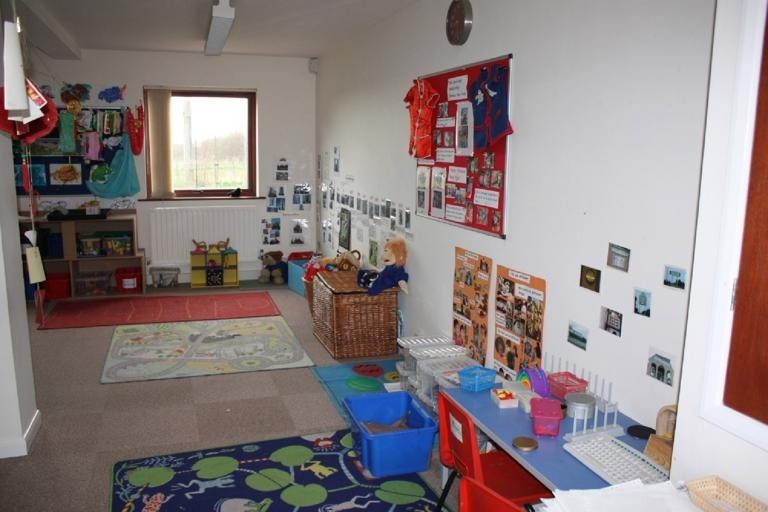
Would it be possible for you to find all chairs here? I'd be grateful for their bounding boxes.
[459,476,525,512]
[433,392,554,511]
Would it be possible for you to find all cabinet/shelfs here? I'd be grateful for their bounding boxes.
[18,208,147,300]
[13,107,123,156]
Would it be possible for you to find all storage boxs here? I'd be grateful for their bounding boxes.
[397,334,451,357]
[115,267,141,292]
[529,398,562,437]
[415,356,480,395]
[343,390,438,476]
[149,266,181,288]
[288,259,309,296]
[431,401,438,424]
[77,235,100,257]
[97,230,133,257]
[549,371,588,401]
[311,270,401,360]
[408,346,472,380]
[435,373,508,394]
[415,392,432,414]
[395,361,416,388]
[39,273,69,298]
[73,271,113,295]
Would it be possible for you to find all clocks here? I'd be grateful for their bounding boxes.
[446,0,472,45]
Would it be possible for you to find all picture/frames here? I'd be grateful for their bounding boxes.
[15,153,91,195]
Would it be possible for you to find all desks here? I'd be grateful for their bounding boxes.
[440,382,670,494]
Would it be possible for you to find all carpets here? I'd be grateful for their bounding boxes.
[109,425,452,512]
[100,314,316,383]
[37,290,281,330]
